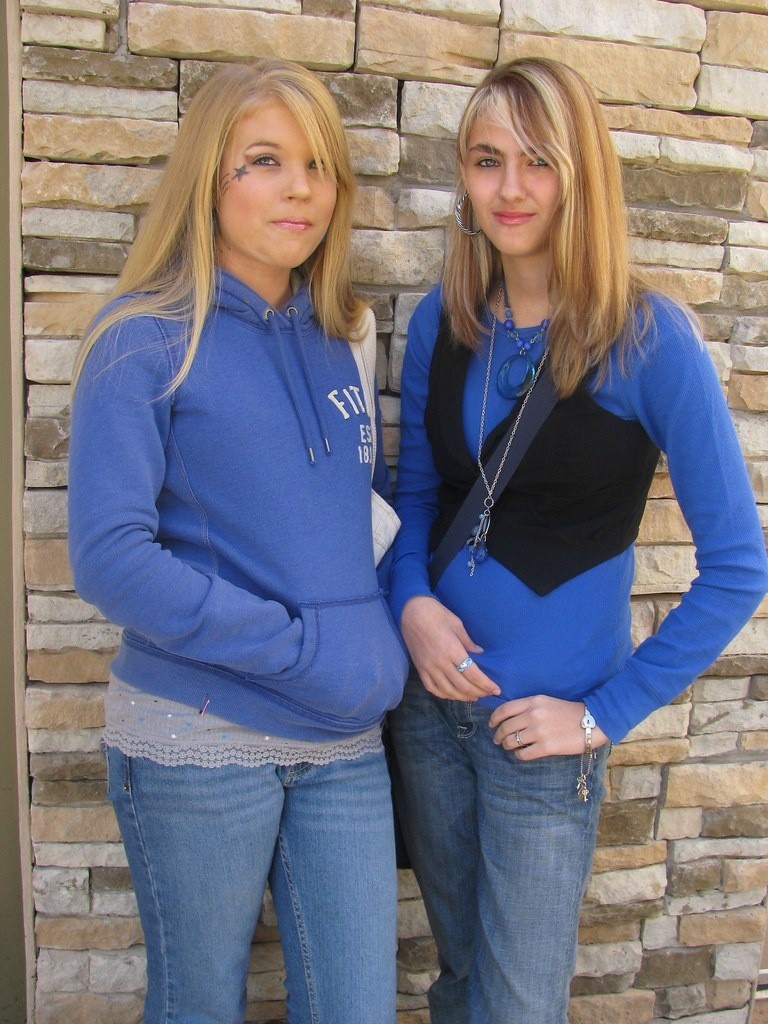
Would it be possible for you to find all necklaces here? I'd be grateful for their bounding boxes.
[467,282,549,577]
[497,264,549,400]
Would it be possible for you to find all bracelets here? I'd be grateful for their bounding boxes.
[577,705,595,802]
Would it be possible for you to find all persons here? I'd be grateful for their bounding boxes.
[387,58,768,1023]
[69,57,412,1024]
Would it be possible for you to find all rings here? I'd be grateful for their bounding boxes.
[457,658,472,672]
[516,732,526,746]
